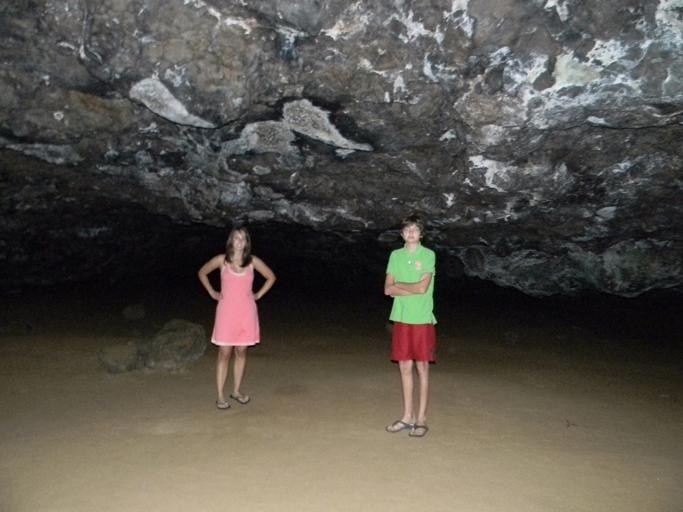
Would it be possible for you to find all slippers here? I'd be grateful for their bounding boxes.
[215,400,231,410]
[230,392,250,405]
[386,419,413,433]
[408,422,429,437]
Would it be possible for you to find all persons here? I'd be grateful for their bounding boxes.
[384,215,438,437]
[197,226,276,409]
